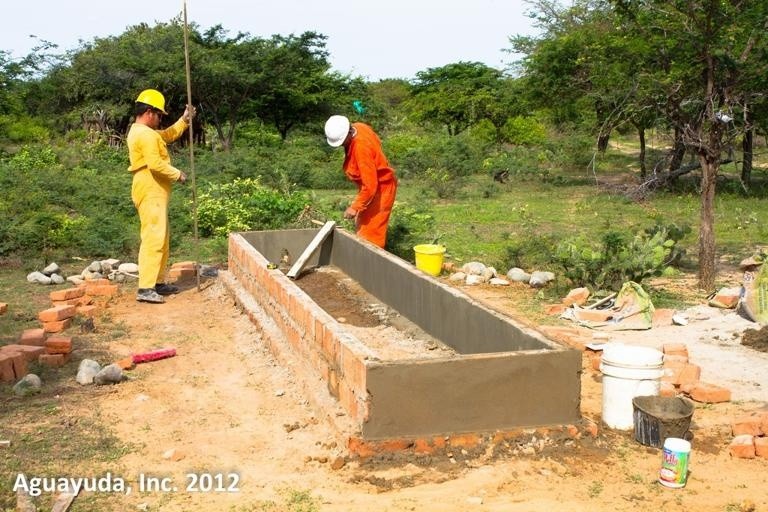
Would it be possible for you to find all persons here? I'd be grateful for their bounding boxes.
[325,115,398,248]
[126,88,197,303]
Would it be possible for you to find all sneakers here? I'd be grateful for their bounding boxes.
[136,283,180,303]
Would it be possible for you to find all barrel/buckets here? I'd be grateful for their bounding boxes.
[412,243,447,275]
[631,396,695,447]
[599,344,665,428]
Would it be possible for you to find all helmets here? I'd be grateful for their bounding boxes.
[325,114,350,148]
[136,89,169,115]
